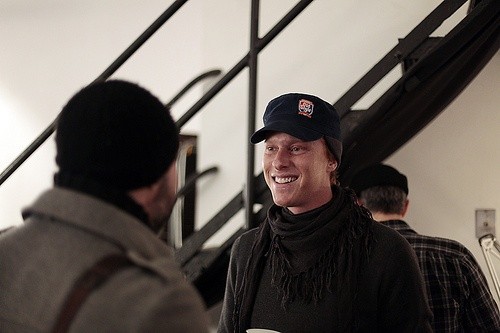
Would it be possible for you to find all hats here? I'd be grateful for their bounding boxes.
[55,79,178,190]
[351,163,408,195]
[250,93,342,141]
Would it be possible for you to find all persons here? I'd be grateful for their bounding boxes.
[0,80,210,333]
[354,164,500,333]
[217,93,435,333]
